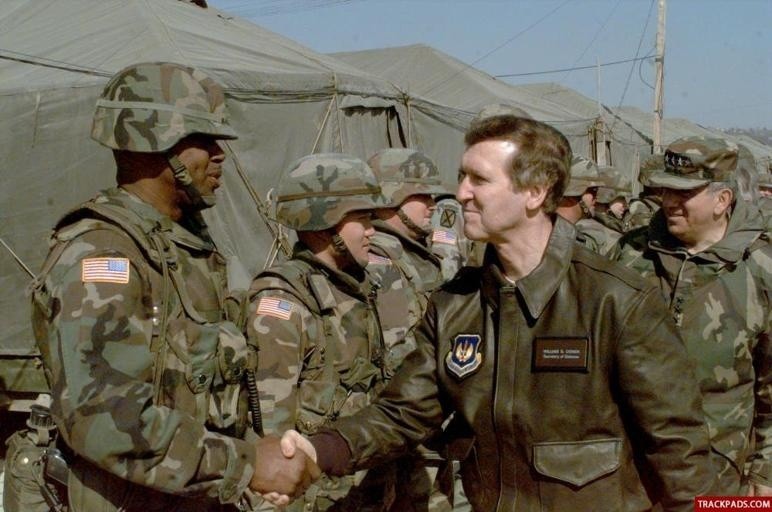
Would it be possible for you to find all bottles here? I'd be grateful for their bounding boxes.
[3,405,63,512]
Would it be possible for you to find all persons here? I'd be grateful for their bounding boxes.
[29,60,322,512]
[252,111,717,511]
[248,151,402,512]
[603,132,771,511]
[366,146,772,511]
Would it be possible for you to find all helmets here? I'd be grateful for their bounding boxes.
[368,149,446,208]
[91,61,238,154]
[563,145,771,203]
[274,153,386,231]
